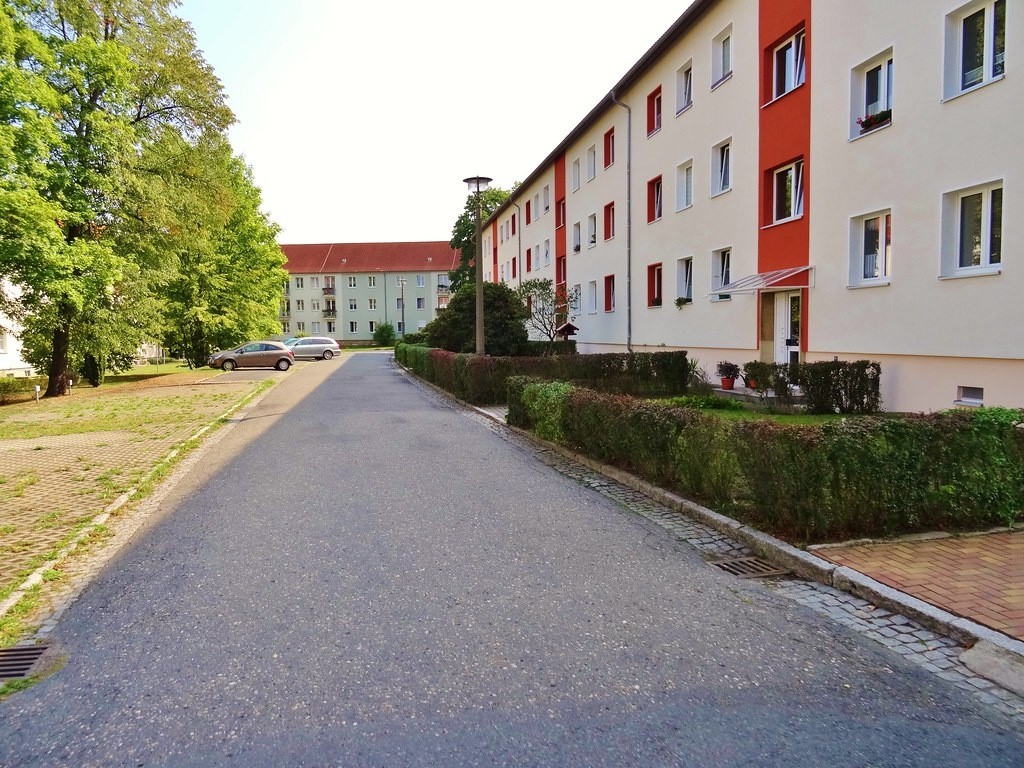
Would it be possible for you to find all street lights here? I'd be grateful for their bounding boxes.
[463,175,494,357]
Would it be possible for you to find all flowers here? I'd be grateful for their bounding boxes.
[854,108,892,130]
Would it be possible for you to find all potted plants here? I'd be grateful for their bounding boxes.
[714,360,770,391]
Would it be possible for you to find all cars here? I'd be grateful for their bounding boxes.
[287,336,341,360]
[207,340,295,371]
[268,338,304,350]
